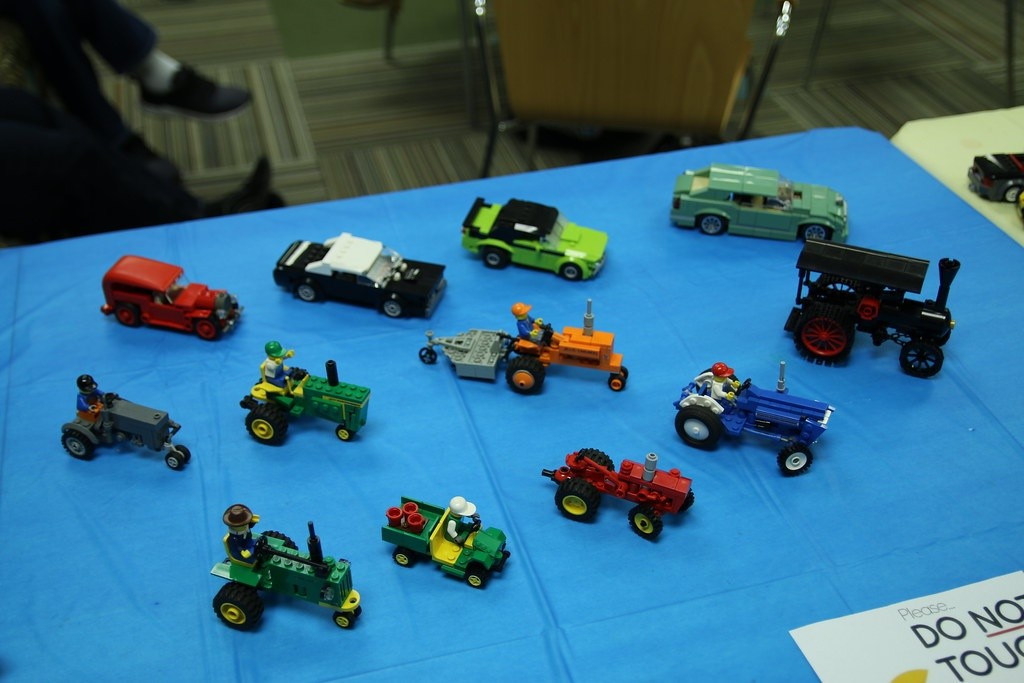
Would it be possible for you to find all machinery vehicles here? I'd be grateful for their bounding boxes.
[543,448,696,538]
[60,374,189,472]
[674,362,835,473]
[416,296,631,396]
[207,503,362,636]
[240,338,371,448]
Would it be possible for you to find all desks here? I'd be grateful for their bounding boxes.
[890,104,1024,250]
[0,126,1024,683]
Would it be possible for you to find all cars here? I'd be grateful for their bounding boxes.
[968,152,1024,203]
[273,233,449,320]
[669,164,848,246]
[98,255,245,342]
[464,196,608,281]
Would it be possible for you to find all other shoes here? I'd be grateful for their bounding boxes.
[139,63,256,119]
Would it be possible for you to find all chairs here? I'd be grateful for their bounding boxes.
[471,0,794,180]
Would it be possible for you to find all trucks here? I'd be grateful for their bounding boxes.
[381,494,508,586]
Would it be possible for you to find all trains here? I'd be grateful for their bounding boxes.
[788,240,960,377]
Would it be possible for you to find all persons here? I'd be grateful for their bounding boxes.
[222,504,259,565]
[77,374,102,416]
[710,360,737,403]
[441,495,479,546]
[513,303,545,340]
[1,0,289,250]
[263,342,296,386]
